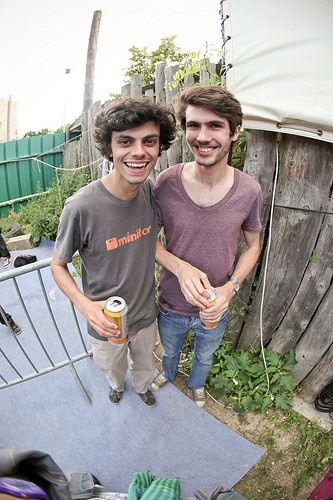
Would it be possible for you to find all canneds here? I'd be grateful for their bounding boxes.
[200,290,217,330]
[104,297,128,346]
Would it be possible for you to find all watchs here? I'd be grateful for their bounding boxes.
[228,279,240,296]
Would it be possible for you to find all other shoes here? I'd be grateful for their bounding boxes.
[192,385,207,407]
[3,258,10,268]
[151,369,169,390]
[139,388,157,408]
[10,323,22,334]
[108,386,123,404]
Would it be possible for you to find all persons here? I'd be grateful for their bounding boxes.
[150,85,262,407]
[0,231,11,268]
[0,448,249,500]
[50,95,177,408]
[0,305,22,335]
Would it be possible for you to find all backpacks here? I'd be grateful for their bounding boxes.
[13,254,37,268]
